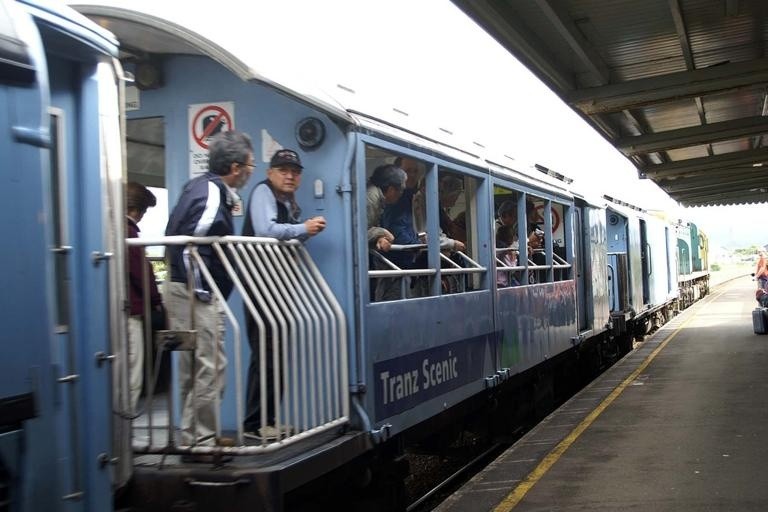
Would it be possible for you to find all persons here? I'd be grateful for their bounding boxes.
[164,130,259,461]
[238,148,326,440]
[751,246,768,313]
[366,155,562,302]
[125,180,162,450]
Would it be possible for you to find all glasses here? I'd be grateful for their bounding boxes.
[275,167,301,176]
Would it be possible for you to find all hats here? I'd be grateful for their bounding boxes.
[440,176,465,195]
[497,199,518,213]
[271,149,306,168]
[126,181,156,208]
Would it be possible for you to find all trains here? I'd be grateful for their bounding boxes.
[0,0,710,511]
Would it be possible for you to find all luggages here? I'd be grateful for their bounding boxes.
[752,306,768,333]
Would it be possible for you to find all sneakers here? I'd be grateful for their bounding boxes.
[181,438,236,464]
[243,422,294,440]
